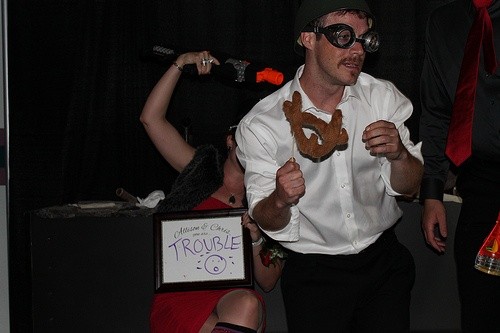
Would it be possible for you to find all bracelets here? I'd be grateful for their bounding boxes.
[251,237,263,247]
[173,62,182,71]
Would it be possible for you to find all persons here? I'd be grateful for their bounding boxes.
[235,1,425,333]
[140,51,286,333]
[420,0,500,333]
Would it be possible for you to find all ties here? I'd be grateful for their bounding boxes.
[445,7,484,166]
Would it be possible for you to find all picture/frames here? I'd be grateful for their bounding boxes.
[153,207,255,293]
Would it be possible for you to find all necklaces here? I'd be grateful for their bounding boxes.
[223,184,244,204]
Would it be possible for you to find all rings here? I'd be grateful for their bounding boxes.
[202,58,212,65]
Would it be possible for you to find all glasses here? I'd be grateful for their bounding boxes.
[312,23,380,53]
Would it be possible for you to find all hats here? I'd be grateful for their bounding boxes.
[296,0,373,47]
